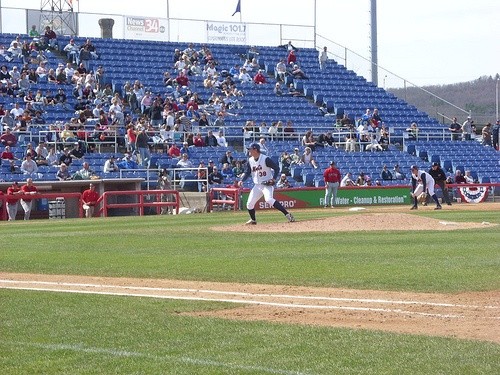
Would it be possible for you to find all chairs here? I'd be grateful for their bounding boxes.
[0,34,500,189]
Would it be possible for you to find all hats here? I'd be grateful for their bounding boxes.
[13,181,18,185]
[431,162,438,166]
[395,164,398,168]
[164,84,196,106]
[71,39,74,42]
[410,165,417,170]
[497,119,500,122]
[330,161,334,165]
[26,153,32,156]
[246,143,260,149]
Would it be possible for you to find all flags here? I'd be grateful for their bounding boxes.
[232,0,240,16]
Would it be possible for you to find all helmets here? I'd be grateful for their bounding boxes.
[486,123,491,126]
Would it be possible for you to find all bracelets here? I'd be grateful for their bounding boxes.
[29,192,30,195]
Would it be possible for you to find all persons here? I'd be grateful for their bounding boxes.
[472,121,477,138]
[323,160,342,208]
[243,118,318,170]
[410,164,442,210]
[454,170,467,184]
[340,172,381,186]
[121,79,246,192]
[20,179,38,220]
[406,123,421,141]
[318,46,329,70]
[317,107,390,152]
[238,143,295,224]
[319,103,331,116]
[393,164,407,180]
[448,117,462,140]
[82,182,100,217]
[422,162,452,206]
[6,182,23,220]
[277,174,293,190]
[1,24,125,182]
[157,168,174,215]
[491,119,500,151]
[381,165,393,181]
[445,177,454,201]
[463,171,475,184]
[164,42,311,97]
[461,116,472,141]
[482,123,492,146]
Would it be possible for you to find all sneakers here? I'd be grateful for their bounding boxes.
[286,213,294,222]
[245,219,256,224]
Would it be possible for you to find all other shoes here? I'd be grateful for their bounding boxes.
[169,212,173,214]
[324,206,327,209]
[434,207,442,210]
[331,206,334,209]
[410,206,418,209]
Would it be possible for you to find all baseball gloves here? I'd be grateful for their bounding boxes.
[261,179,274,186]
